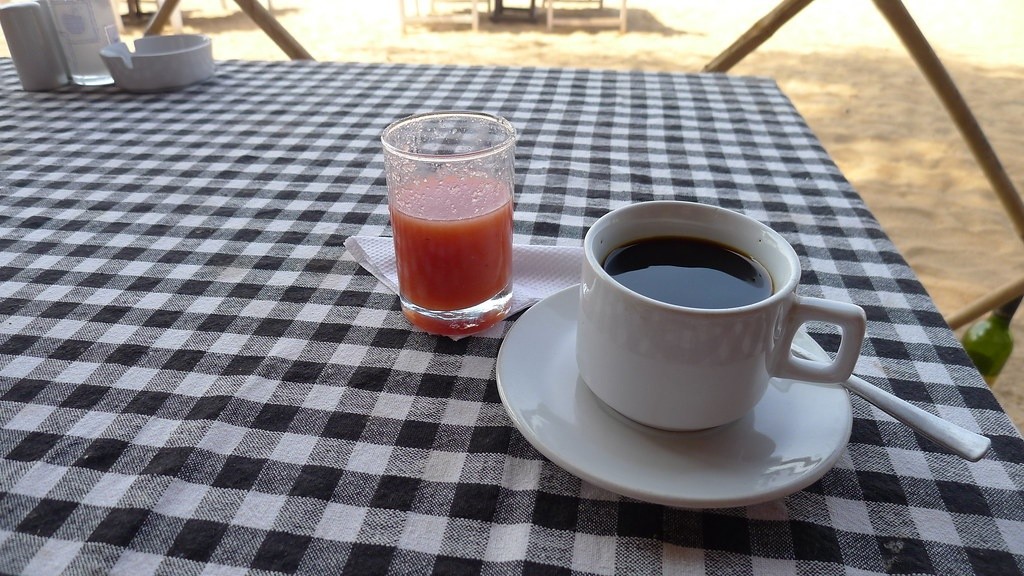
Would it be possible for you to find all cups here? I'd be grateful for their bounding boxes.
[381,109,518,336]
[576,200,868,433]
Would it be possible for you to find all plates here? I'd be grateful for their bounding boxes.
[496,279,855,511]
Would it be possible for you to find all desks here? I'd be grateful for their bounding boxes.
[0,55,1024,576]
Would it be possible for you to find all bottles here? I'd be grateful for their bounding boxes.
[962,293,1023,392]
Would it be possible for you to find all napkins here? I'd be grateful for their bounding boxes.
[342,235,585,340]
